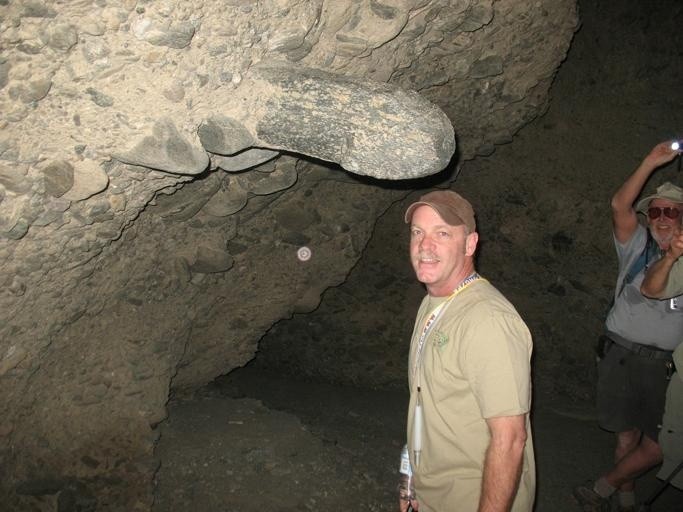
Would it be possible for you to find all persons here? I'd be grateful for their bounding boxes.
[572,139,682,512]
[397,189,537,511]
[640,226,683,512]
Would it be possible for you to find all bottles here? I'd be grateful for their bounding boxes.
[672,139,683,150]
[397,443,416,500]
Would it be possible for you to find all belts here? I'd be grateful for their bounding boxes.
[603,329,674,360]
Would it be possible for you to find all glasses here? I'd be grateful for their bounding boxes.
[646,207,683,219]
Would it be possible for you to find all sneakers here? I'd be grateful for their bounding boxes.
[572,479,616,512]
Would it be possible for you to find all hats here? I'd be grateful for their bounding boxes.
[404,190,476,233]
[635,181,683,216]
